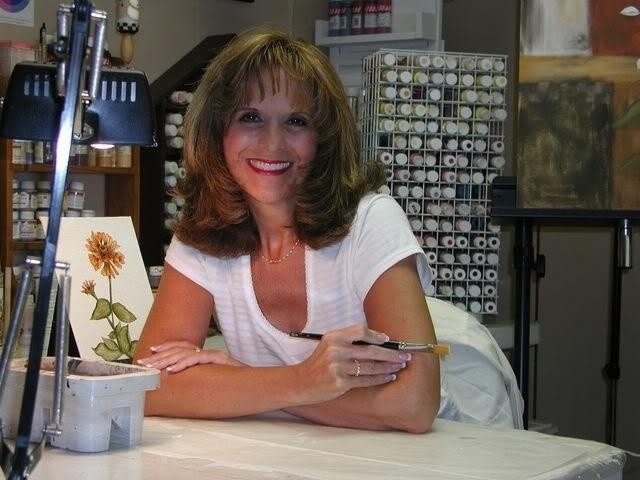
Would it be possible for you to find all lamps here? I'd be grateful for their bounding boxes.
[0,0,159,479]
[116,1,141,65]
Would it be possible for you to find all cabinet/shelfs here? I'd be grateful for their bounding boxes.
[360,48,508,316]
[0,34,142,309]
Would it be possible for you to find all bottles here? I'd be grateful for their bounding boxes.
[12,179,97,240]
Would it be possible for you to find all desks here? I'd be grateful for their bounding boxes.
[0,411,627,480]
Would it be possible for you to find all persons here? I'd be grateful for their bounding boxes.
[132,24,463,434]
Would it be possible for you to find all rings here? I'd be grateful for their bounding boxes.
[352,358,363,379]
[255,238,302,265]
[193,347,201,354]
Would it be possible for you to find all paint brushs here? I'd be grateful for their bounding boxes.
[290,332,450,355]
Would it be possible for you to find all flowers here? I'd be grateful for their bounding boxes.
[80,228,140,362]
